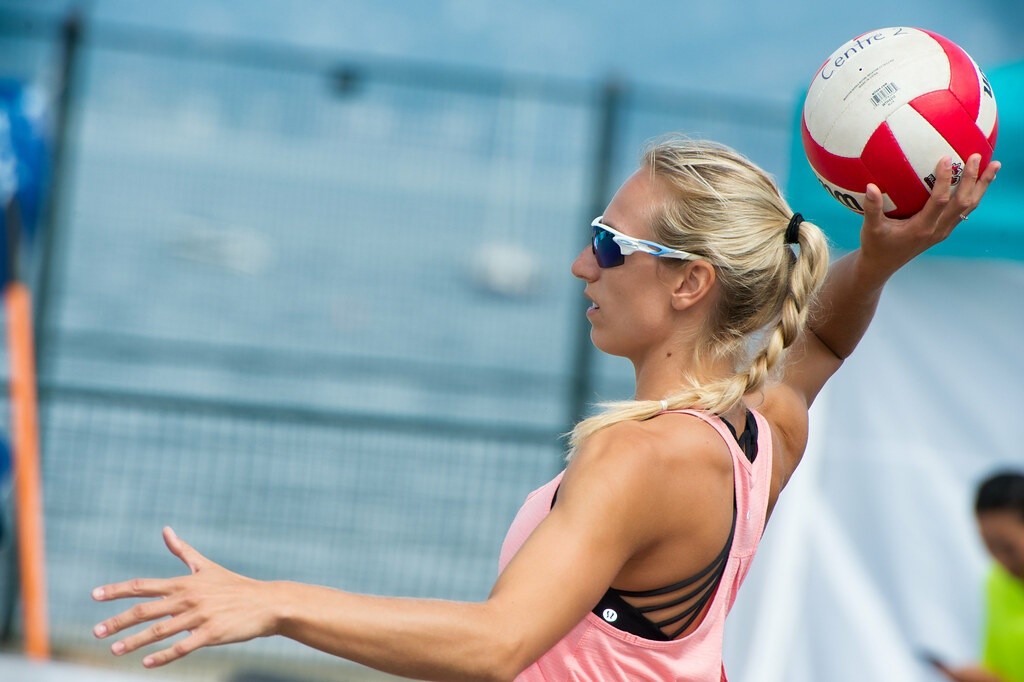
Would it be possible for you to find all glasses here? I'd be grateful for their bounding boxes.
[590,216,709,268]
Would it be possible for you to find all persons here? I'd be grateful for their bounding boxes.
[91,138,1001,681]
[947,474,1024,682]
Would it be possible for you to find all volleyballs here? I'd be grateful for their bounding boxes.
[802,26,998,220]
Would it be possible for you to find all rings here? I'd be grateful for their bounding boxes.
[960,212,969,221]
